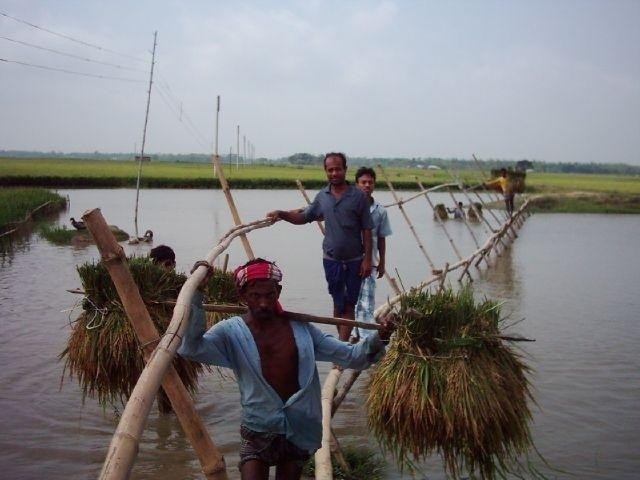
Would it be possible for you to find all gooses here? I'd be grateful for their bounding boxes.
[138,230,153,242]
[69,217,87,231]
[128,232,140,244]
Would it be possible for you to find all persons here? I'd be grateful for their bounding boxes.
[447,201,467,221]
[149,244,187,415]
[482,167,516,220]
[287,164,394,345]
[265,151,376,373]
[175,257,402,480]
